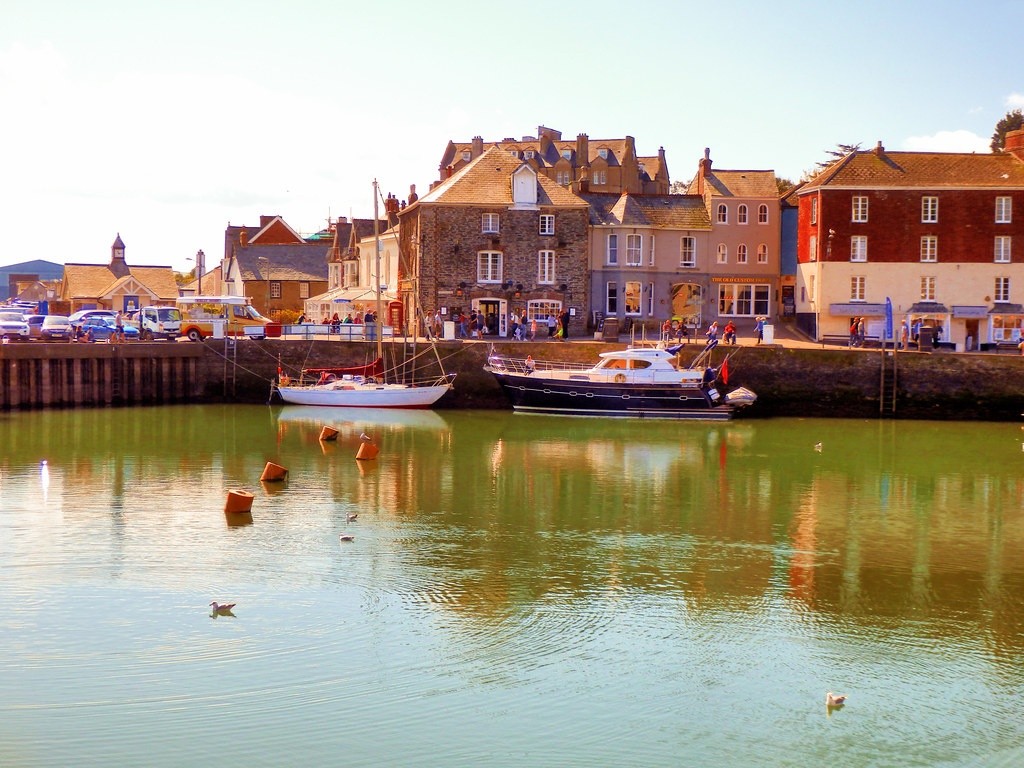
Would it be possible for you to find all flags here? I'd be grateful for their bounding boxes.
[721,360,729,384]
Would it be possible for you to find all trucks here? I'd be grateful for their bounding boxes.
[122,306,183,340]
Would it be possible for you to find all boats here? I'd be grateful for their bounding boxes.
[275,404,451,435]
[483,323,757,421]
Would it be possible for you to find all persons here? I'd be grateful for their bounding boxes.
[297,312,307,324]
[531,319,537,341]
[754,318,768,344]
[913,317,923,349]
[111,310,125,341]
[331,313,343,333]
[457,309,486,340]
[434,310,443,339]
[322,318,332,324]
[900,320,910,337]
[931,321,945,350]
[851,316,868,348]
[508,308,528,341]
[724,320,737,345]
[706,321,719,344]
[662,319,689,340]
[344,313,353,323]
[524,355,535,376]
[424,310,433,341]
[353,312,364,324]
[364,309,377,323]
[546,311,570,340]
[75,326,97,343]
[1016,332,1024,353]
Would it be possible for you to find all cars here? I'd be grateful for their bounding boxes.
[41,316,73,342]
[0,312,31,342]
[0,301,46,340]
[82,317,140,342]
[68,309,116,328]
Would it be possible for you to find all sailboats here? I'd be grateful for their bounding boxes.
[276,177,458,408]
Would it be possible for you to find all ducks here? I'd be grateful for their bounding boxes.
[826,702,844,717]
[815,442,823,447]
[826,692,846,705]
[339,533,354,541]
[345,512,357,520]
[209,601,236,610]
[208,609,236,618]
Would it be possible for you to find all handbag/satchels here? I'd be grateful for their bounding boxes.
[849,323,857,335]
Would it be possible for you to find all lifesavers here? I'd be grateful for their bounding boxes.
[281,377,289,386]
[615,373,626,382]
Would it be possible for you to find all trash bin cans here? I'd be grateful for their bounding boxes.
[919,327,934,352]
[604,318,619,342]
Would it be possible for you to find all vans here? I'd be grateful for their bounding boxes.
[175,296,273,342]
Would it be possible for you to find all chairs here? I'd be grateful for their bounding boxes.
[996,340,1022,355]
[722,333,736,344]
[822,334,879,350]
[191,314,220,318]
